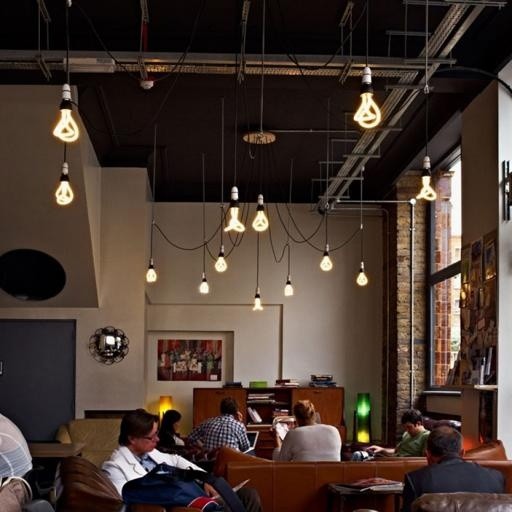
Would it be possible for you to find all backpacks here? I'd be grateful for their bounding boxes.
[121,462,246,512]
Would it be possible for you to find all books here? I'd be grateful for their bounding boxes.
[247,393,276,403]
[310,375,336,388]
[275,379,299,388]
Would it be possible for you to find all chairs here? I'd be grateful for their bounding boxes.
[1,409,511,511]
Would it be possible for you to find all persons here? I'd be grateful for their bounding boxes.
[272,400,342,462]
[158,410,186,446]
[159,341,221,380]
[366,409,431,457]
[0,413,36,488]
[101,408,262,512]
[404,425,505,512]
[185,396,256,457]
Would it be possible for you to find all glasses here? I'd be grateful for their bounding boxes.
[136,430,161,441]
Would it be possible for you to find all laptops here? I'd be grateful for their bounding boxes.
[244,431,259,453]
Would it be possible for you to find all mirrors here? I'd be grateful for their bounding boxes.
[87,324,131,368]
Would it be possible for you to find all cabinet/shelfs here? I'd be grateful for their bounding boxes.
[191,385,346,457]
[458,382,497,452]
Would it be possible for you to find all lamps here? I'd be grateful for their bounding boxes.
[351,392,373,443]
[157,393,174,430]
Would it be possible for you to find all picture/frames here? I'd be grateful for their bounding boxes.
[151,333,225,387]
